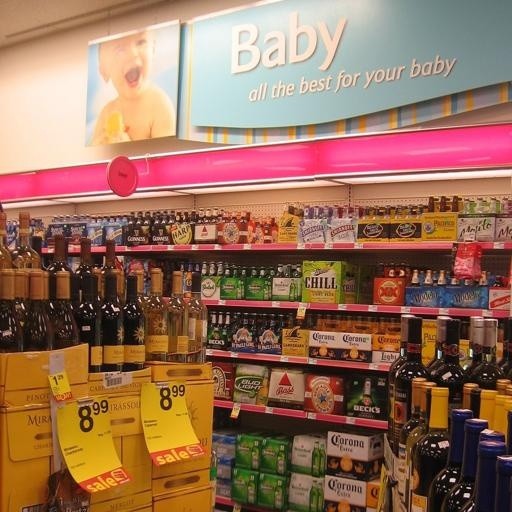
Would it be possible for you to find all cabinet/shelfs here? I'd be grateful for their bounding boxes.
[0,194,512,510]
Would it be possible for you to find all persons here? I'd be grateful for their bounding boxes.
[89,29,179,145]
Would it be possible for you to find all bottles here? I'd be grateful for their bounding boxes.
[0,205,209,374]
[245,474,257,504]
[273,479,285,510]
[209,259,301,280]
[254,368,271,406]
[376,260,505,289]
[208,196,512,226]
[235,278,298,301]
[310,439,326,477]
[276,441,286,473]
[387,315,512,512]
[352,378,378,417]
[308,480,325,511]
[249,440,261,470]
[209,312,402,350]
